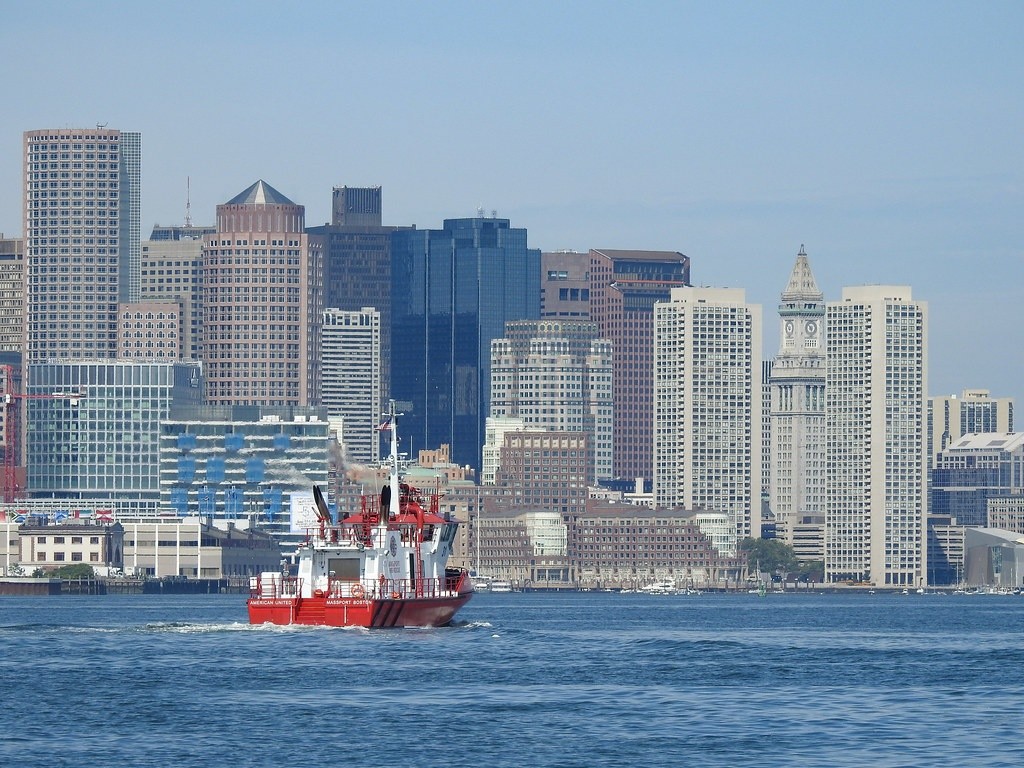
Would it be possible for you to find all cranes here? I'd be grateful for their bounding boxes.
[1,364,80,503]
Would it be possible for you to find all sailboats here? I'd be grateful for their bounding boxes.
[916,557,1024,597]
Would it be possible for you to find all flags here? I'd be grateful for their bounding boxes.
[55,511,68,520]
[74,510,92,519]
[96,509,112,521]
[11,510,28,521]
[375,417,392,429]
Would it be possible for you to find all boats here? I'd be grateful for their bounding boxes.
[474,582,512,594]
[902,588,909,594]
[245,399,478,628]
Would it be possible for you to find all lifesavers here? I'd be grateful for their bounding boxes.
[351,583,364,597]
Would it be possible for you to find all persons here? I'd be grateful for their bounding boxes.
[379,574,386,597]
